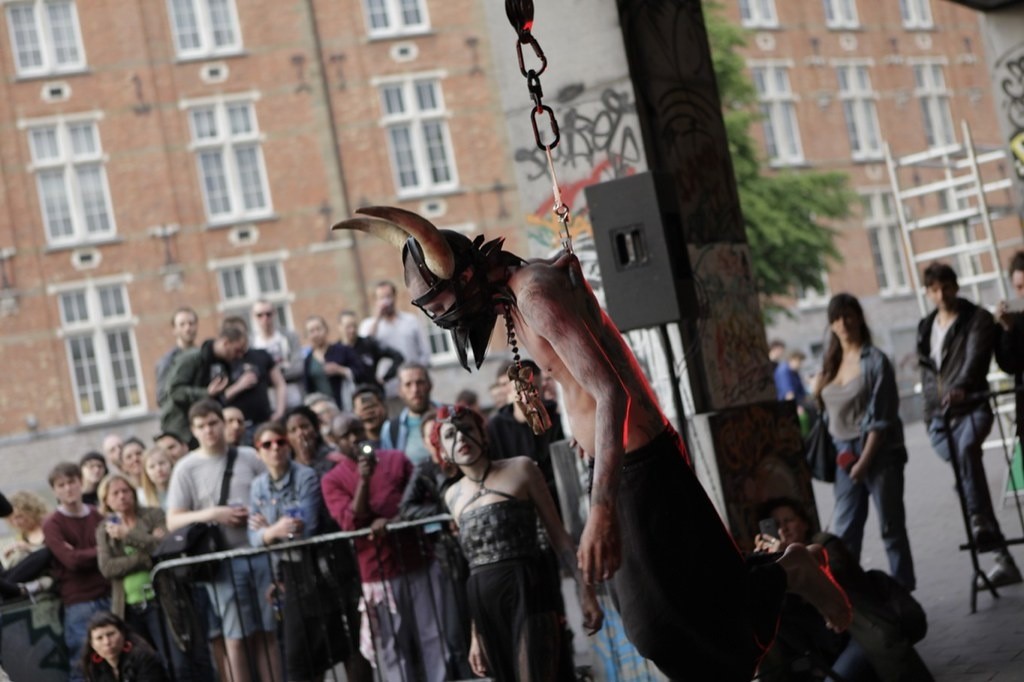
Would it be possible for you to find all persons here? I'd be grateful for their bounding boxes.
[753,497,875,682]
[809,292,917,592]
[403,231,851,682]
[774,350,810,414]
[993,251,1024,474]
[0,282,574,682]
[916,262,1022,589]
[768,341,785,370]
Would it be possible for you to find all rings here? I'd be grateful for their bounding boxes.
[771,538,776,544]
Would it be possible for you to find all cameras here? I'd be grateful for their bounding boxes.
[359,440,377,469]
[209,362,224,381]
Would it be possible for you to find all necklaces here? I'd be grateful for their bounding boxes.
[500,266,552,435]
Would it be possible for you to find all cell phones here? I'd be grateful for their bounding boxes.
[758,517,780,540]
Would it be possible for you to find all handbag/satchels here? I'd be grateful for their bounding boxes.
[182,442,238,586]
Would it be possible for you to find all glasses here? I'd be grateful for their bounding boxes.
[255,438,292,447]
[254,312,272,317]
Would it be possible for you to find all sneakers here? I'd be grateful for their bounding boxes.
[976,563,1024,594]
[957,526,1004,547]
